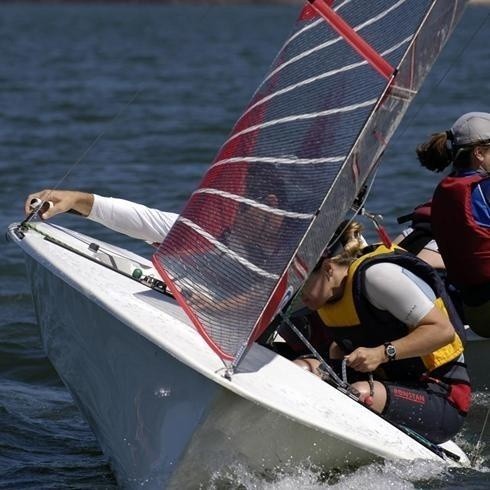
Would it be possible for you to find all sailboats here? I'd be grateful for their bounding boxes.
[5,0,490,490]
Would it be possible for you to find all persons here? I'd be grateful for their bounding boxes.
[22,161,367,366]
[283,220,472,446]
[414,111,490,339]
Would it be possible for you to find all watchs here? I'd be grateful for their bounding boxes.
[383,341,396,363]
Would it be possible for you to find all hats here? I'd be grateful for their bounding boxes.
[451,112,490,146]
